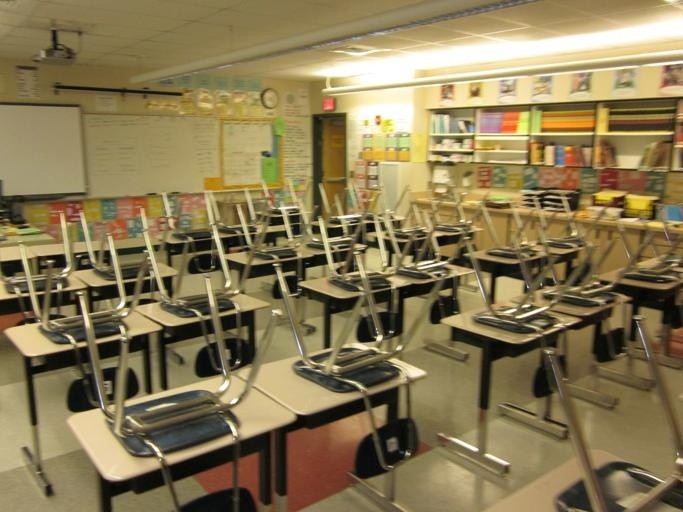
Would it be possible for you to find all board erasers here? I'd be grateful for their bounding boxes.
[172,191,180,194]
[147,192,157,195]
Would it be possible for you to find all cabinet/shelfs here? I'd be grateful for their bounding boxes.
[569,222,639,284]
[639,231,683,308]
[456,209,509,270]
[412,204,459,257]
[423,97,682,173]
[508,214,566,283]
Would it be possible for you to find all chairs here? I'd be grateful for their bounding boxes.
[316,181,367,237]
[617,216,683,330]
[239,204,317,298]
[316,218,418,347]
[232,186,290,251]
[381,203,440,266]
[356,179,409,231]
[534,198,611,287]
[80,209,171,310]
[645,214,683,264]
[544,315,683,512]
[462,239,594,397]
[480,208,557,295]
[535,230,655,362]
[261,180,300,230]
[432,193,486,265]
[16,234,149,412]
[273,243,450,480]
[146,219,269,379]
[385,216,473,325]
[2,216,80,322]
[76,274,283,512]
[163,188,234,274]
[300,193,377,274]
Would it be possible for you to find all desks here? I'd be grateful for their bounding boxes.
[384,261,477,334]
[443,304,582,411]
[366,222,429,266]
[164,226,228,266]
[1,270,87,317]
[464,246,548,305]
[297,269,411,350]
[71,238,162,270]
[10,306,163,425]
[598,264,682,343]
[430,218,483,259]
[296,229,367,285]
[237,343,427,497]
[135,288,270,392]
[512,283,635,354]
[638,251,683,322]
[0,244,71,274]
[312,216,364,240]
[256,204,310,226]
[223,243,303,300]
[524,235,593,290]
[358,212,404,233]
[227,222,291,248]
[65,372,296,512]
[0,222,54,246]
[74,258,180,314]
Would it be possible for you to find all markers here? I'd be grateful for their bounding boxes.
[128,194,136,197]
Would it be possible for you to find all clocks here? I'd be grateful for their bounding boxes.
[261,88,279,109]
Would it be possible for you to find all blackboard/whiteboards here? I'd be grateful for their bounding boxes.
[1,101,87,199]
[66,116,284,203]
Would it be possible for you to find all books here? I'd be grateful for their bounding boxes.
[479,109,529,133]
[431,113,474,134]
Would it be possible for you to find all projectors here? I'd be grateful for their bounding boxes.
[31,48,79,67]
